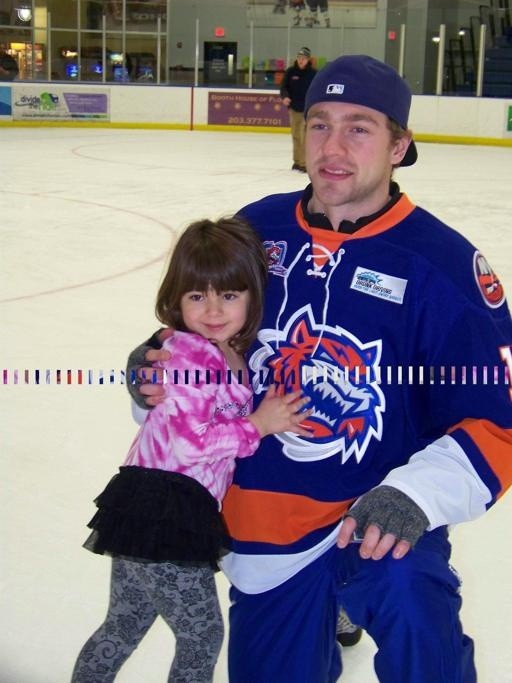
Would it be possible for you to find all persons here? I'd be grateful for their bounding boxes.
[71,213,319,680]
[279,46,320,172]
[126,55,510,680]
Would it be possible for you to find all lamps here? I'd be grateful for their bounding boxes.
[14,0,33,21]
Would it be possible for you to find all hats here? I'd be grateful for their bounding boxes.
[303,54,418,168]
[297,46,311,57]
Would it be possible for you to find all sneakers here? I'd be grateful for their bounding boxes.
[336,604,362,647]
[292,164,306,173]
